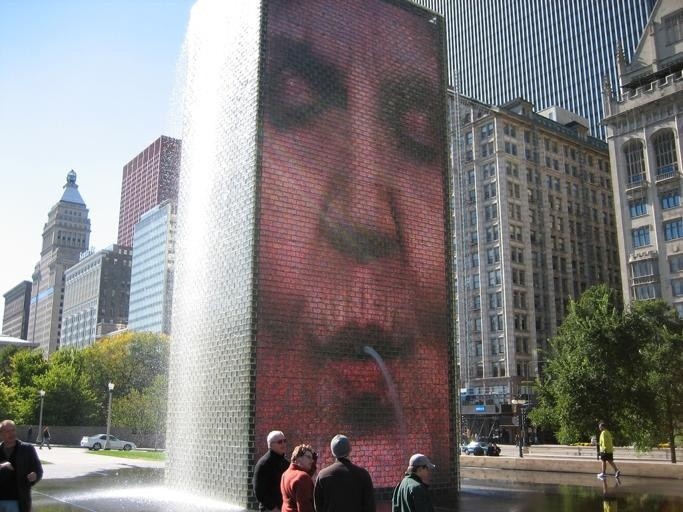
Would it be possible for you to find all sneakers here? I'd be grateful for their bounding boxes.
[597,472,606,477]
[614,471,621,478]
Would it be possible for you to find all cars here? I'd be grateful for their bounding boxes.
[80,434,137,452]
[459,442,499,455]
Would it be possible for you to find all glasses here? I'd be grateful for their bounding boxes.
[272,439,287,444]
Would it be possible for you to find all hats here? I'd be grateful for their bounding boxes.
[331,435,352,458]
[409,454,435,468]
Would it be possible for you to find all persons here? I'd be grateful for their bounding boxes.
[308,434,377,510]
[389,452,439,511]
[0,418,41,510]
[251,429,288,511]
[38,424,51,451]
[597,476,622,511]
[24,422,33,444]
[596,424,621,479]
[246,0,449,489]
[462,428,520,456]
[277,442,318,511]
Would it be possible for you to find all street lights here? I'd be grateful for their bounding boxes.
[509,395,530,459]
[36,389,46,446]
[103,380,118,451]
